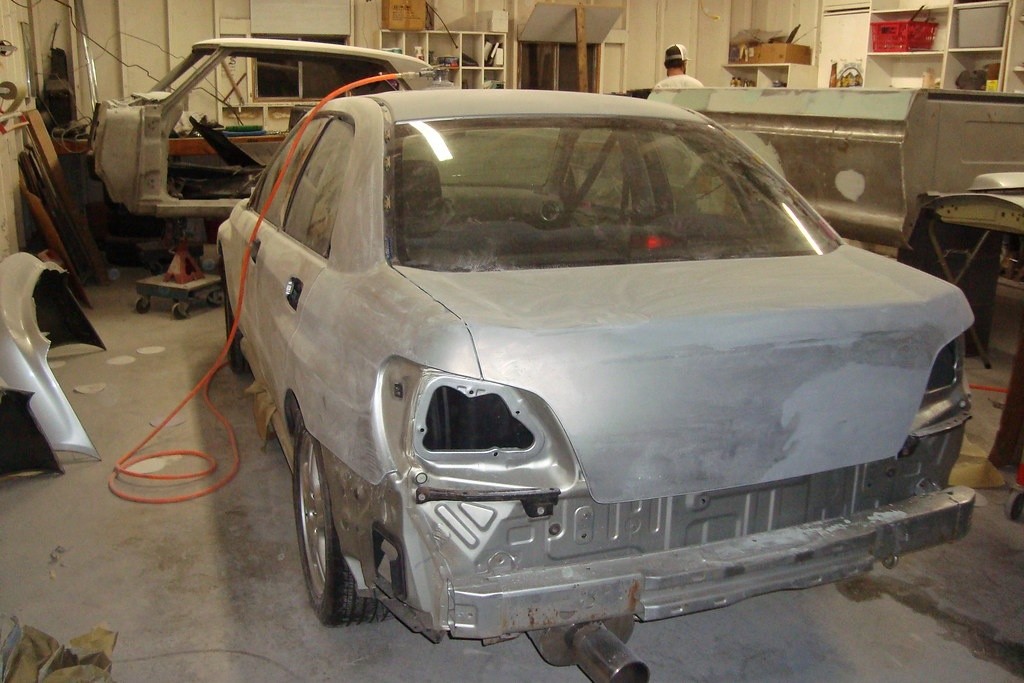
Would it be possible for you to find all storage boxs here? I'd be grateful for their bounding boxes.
[754,45,811,64]
[382,0,426,31]
[477,10,509,33]
[871,21,937,51]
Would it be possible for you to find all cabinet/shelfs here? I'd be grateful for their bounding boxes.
[379,29,506,89]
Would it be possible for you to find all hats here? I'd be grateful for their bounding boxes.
[665,44,692,61]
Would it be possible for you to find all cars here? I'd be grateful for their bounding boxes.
[90,37,459,216]
[215,89,978,683]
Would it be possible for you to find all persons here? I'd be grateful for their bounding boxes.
[652,44,704,89]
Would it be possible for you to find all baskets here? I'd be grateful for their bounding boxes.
[870,21,939,52]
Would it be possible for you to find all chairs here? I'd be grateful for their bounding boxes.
[402,160,457,230]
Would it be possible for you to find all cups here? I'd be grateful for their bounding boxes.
[921,72,935,89]
